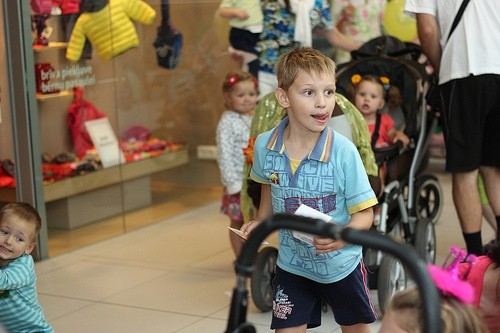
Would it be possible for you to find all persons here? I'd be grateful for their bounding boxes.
[214,0,500,333]
[0,203,54,333]
[241,47,379,332]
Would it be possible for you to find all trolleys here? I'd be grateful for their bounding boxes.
[224,213,442,333]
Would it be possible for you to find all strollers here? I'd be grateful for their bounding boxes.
[332,34,444,224]
[237,84,437,320]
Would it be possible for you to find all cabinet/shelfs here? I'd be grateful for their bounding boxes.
[34,42,75,103]
